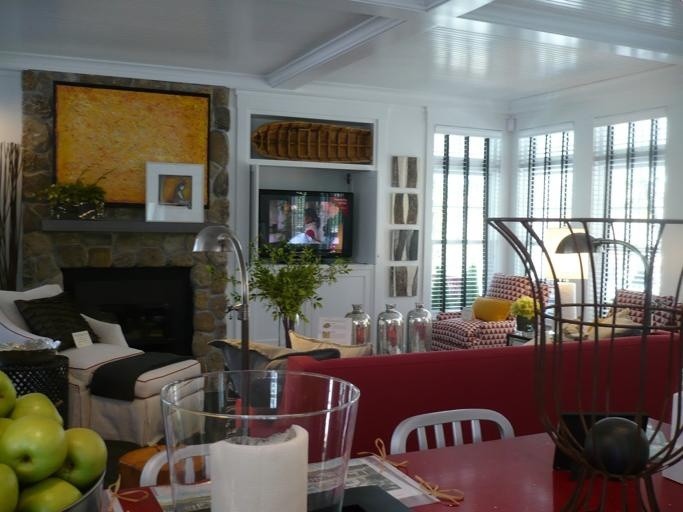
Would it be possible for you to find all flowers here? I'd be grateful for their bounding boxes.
[511,295,540,320]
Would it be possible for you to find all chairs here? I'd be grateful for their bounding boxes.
[431,281,549,352]
[140,441,210,487]
[390,409,515,455]
[0,284,144,427]
[587,303,682,341]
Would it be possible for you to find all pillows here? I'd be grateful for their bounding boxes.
[13,291,98,353]
[587,289,675,340]
[288,329,373,359]
[471,273,532,323]
[206,339,340,426]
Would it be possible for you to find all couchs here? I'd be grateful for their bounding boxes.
[235,329,683,464]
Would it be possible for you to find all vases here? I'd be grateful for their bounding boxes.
[517,316,536,332]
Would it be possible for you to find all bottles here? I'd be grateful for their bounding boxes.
[345,302,433,355]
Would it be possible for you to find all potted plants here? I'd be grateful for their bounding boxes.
[25,167,115,220]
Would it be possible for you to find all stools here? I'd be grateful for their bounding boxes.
[89,352,204,446]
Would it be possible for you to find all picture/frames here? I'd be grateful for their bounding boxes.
[317,316,353,348]
[145,162,204,224]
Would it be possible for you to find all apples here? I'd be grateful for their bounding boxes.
[15,476,84,512]
[0,416,16,440]
[0,371,17,417]
[0,463,19,512]
[6,392,65,429]
[55,427,109,487]
[0,413,69,485]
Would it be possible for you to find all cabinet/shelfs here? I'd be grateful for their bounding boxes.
[248,263,374,346]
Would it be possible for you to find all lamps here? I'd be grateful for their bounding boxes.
[544,227,588,331]
[555,233,652,335]
[193,225,249,437]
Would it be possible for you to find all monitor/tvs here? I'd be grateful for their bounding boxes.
[259,189,354,264]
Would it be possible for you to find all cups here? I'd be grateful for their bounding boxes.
[160,370,361,512]
